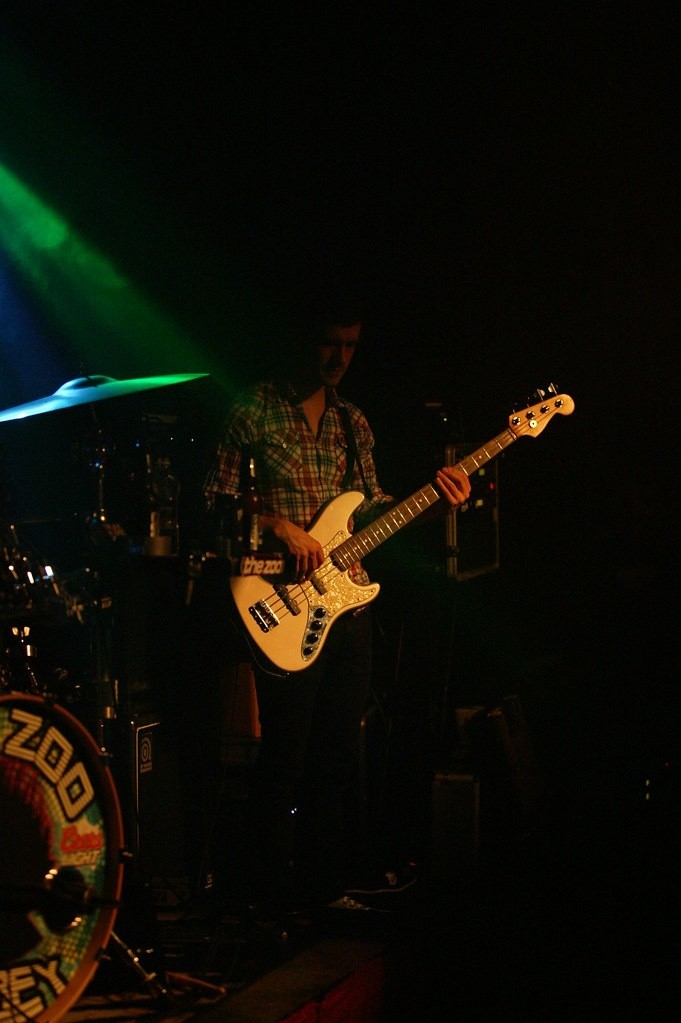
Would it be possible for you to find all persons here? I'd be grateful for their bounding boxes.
[211,294,471,950]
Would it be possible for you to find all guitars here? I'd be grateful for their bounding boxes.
[219,379,577,680]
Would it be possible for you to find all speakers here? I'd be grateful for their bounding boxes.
[132,718,224,890]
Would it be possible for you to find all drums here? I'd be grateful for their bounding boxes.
[1,686,133,1023]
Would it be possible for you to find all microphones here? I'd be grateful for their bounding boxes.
[142,412,192,425]
[37,887,120,915]
[246,458,259,551]
